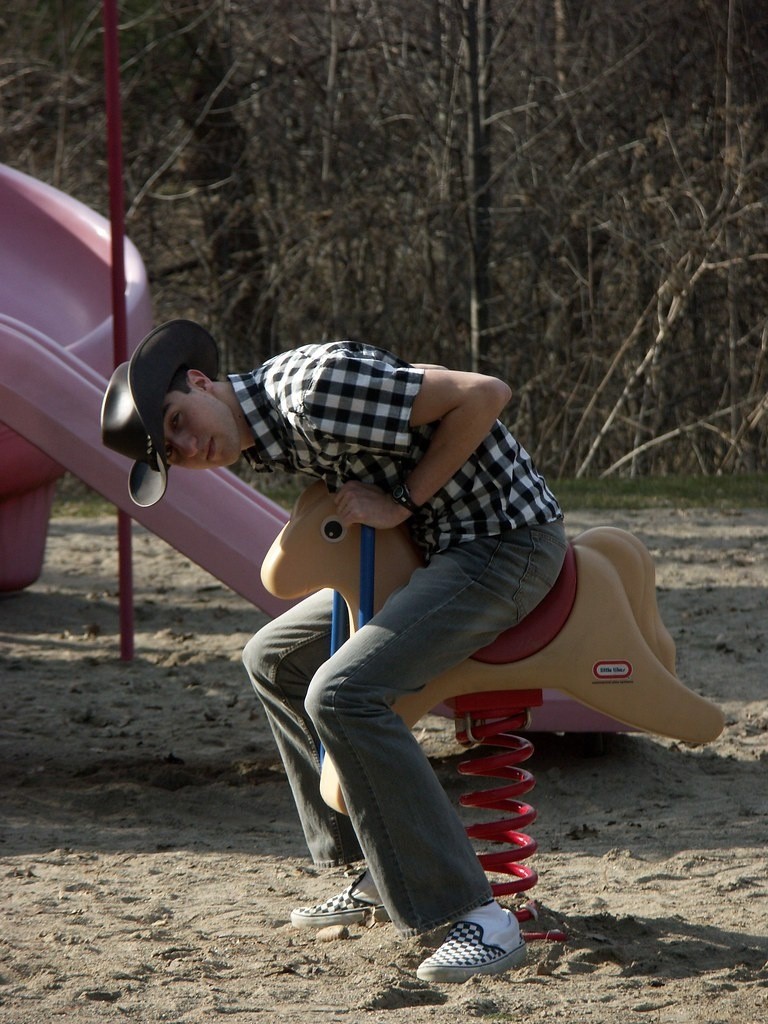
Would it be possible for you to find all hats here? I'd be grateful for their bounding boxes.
[102,319,219,508]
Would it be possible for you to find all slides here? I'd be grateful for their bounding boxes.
[0,161,631,737]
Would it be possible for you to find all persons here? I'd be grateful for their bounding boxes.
[100,321,566,985]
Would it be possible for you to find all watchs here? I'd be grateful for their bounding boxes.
[390,483,422,514]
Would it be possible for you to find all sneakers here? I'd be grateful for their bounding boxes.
[290,871,390,928]
[417,908,527,982]
